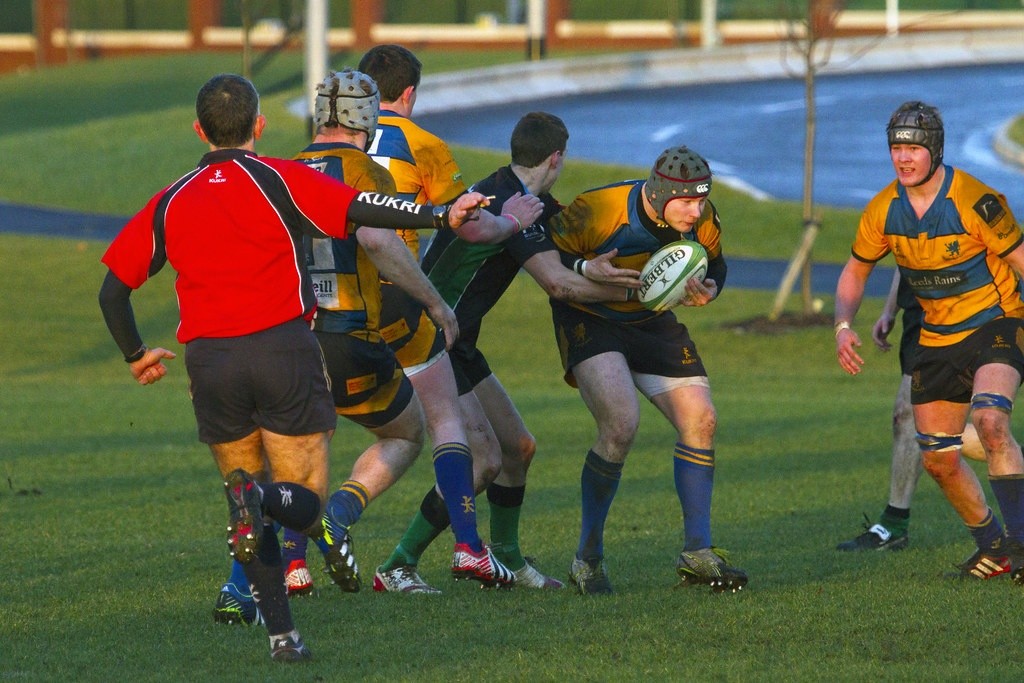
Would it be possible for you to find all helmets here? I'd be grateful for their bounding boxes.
[315,68,381,154]
[888,101,944,187]
[644,145,713,217]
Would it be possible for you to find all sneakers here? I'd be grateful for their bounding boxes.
[373,565,442,595]
[938,544,1011,582]
[284,559,313,595]
[1011,555,1024,585]
[308,509,363,593]
[510,557,568,590]
[225,467,265,563]
[836,512,909,552]
[270,636,312,663]
[212,583,265,627]
[674,543,749,594]
[451,540,516,591]
[569,552,613,597]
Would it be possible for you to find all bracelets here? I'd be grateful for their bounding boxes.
[124,343,146,363]
[501,212,522,234]
[832,320,850,334]
[624,287,634,300]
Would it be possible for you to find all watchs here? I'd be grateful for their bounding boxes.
[433,203,446,229]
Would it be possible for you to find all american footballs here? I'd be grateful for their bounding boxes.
[637,239,709,312]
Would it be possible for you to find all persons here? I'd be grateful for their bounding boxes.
[374,113,648,594]
[97,75,492,659]
[287,67,461,594]
[827,102,1024,585]
[358,43,545,593]
[547,144,748,595]
